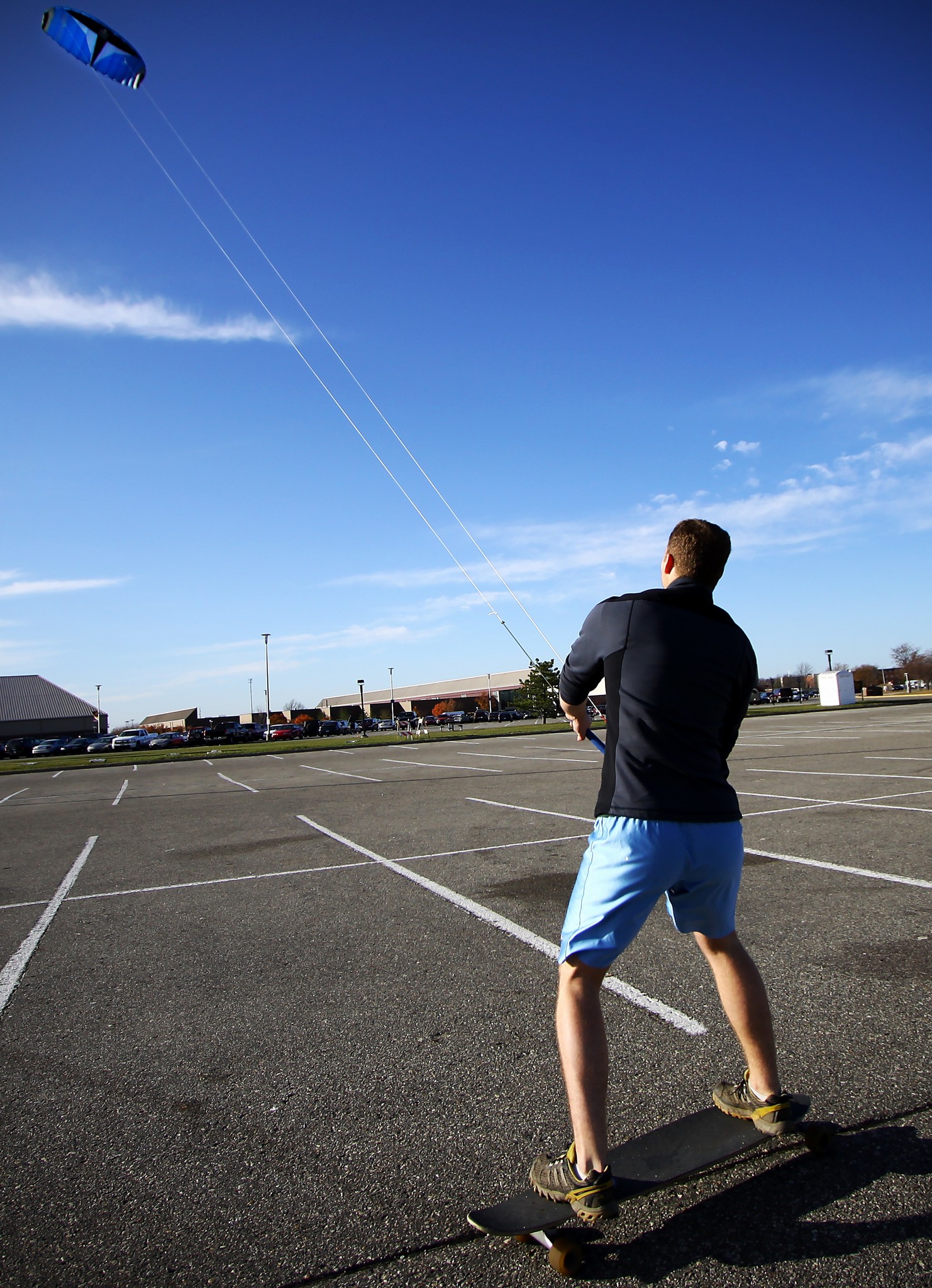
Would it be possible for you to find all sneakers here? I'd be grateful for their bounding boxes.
[713,1069,794,1137]
[529,1139,619,1223]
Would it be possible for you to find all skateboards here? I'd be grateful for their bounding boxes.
[468,1093,811,1275]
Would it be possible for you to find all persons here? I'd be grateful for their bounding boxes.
[529,518,793,1222]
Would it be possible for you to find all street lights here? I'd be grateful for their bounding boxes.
[388,667,394,720]
[261,634,272,741]
[94,685,102,734]
[248,678,253,713]
[357,679,367,736]
[486,673,491,713]
[904,673,909,694]
[825,649,833,671]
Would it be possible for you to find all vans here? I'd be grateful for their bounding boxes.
[891,679,932,691]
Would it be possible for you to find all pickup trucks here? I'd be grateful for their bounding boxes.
[111,728,158,753]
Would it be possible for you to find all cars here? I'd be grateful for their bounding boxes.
[379,719,396,731]
[542,707,565,719]
[58,737,98,755]
[147,719,364,751]
[434,709,538,726]
[87,737,117,754]
[586,705,606,715]
[749,686,820,705]
[422,716,436,727]
[32,738,72,757]
[0,744,6,760]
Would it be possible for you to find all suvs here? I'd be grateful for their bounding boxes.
[4,737,45,759]
[359,717,381,732]
[769,687,794,704]
[394,711,421,730]
